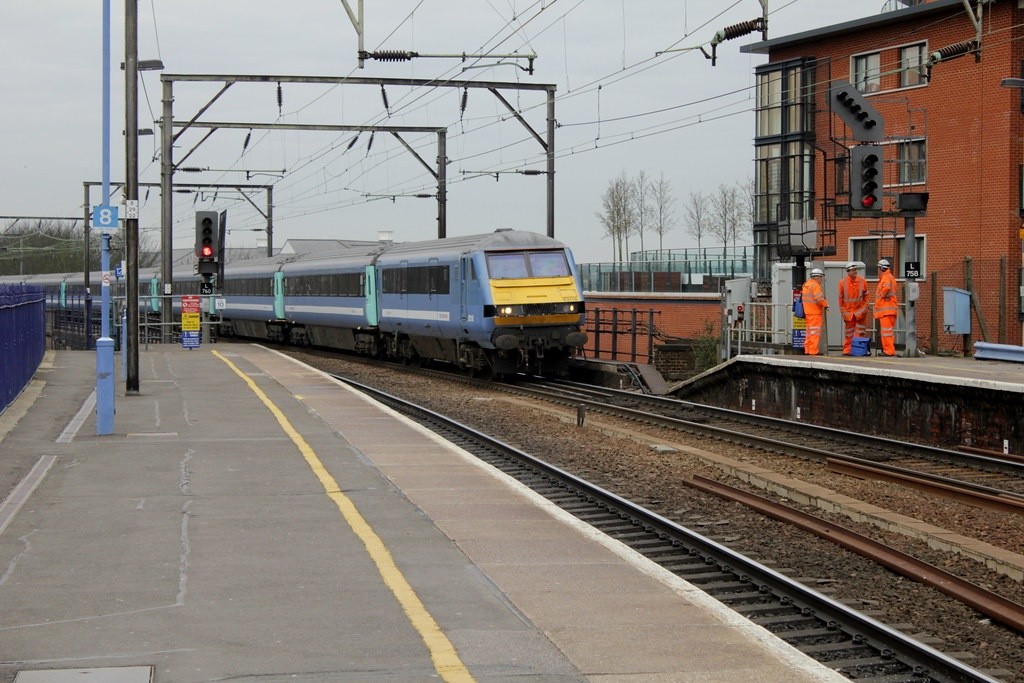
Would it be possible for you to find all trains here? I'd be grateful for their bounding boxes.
[42,226,586,386]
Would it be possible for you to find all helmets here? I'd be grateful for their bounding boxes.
[811,269,825,277]
[846,263,857,272]
[877,259,890,269]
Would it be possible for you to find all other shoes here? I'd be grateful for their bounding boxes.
[879,352,889,356]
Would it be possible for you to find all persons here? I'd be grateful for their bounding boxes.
[838,263,870,356]
[801,269,830,356]
[873,259,898,356]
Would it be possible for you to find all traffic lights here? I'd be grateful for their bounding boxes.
[201,218,212,256]
[861,153,879,209]
[838,89,876,129]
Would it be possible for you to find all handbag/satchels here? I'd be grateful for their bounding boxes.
[795,298,805,317]
[850,337,872,356]
